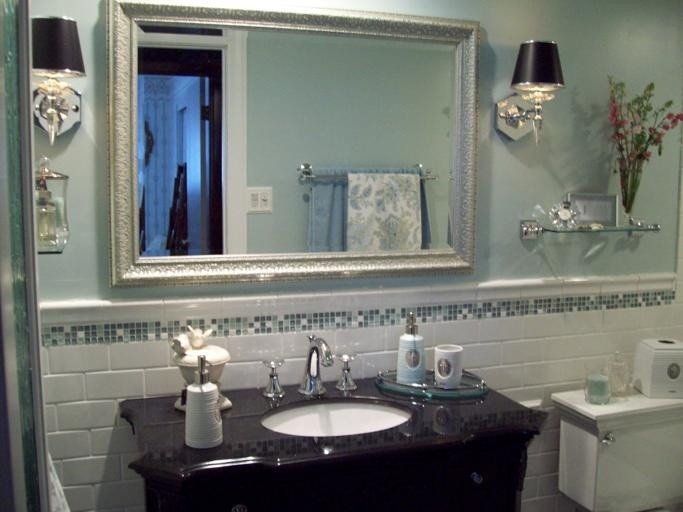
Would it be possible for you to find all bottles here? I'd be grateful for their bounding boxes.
[609,350,630,396]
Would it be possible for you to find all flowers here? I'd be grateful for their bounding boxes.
[602,74,683,215]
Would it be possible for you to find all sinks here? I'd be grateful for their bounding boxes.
[261,400,410,437]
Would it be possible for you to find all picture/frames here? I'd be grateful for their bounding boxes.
[566,192,618,229]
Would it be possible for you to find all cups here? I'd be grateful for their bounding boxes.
[434,345,463,388]
[583,364,610,406]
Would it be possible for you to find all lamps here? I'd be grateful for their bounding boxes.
[32,16,86,147]
[494,43,565,142]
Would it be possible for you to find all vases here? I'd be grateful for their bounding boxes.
[617,158,644,228]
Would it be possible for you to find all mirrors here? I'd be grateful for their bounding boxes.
[105,1,481,286]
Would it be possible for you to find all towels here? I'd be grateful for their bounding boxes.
[345,173,421,251]
[306,166,431,253]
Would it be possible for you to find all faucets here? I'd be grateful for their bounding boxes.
[297,335,334,396]
